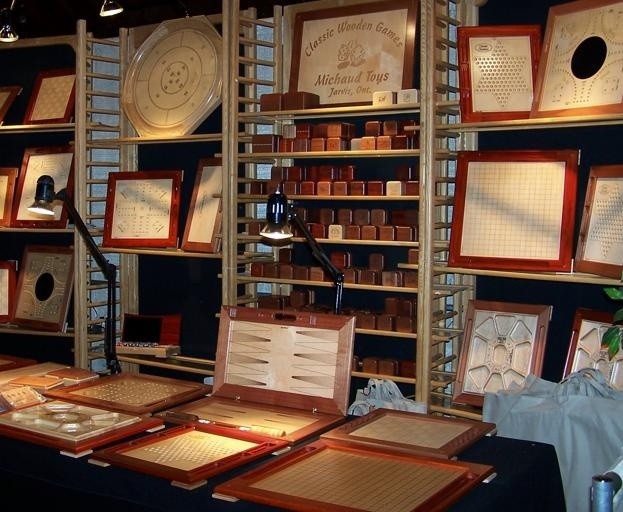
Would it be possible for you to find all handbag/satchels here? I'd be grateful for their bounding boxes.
[356,378,427,414]
[482,367,622,512]
